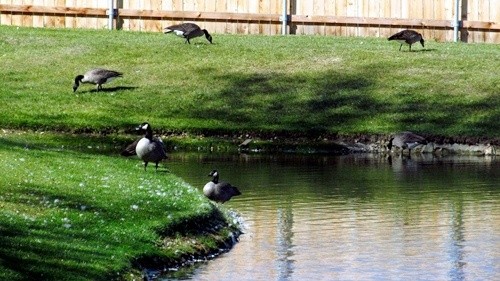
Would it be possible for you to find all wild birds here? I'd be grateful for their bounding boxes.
[387,30,424,52]
[135,121,168,171]
[203,168,242,207]
[164,23,213,45]
[387,132,427,157]
[72,68,124,93]
